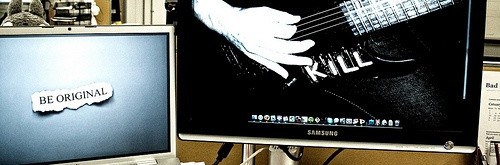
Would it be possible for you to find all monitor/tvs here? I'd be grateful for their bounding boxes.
[175,0,487,155]
[0,25,178,165]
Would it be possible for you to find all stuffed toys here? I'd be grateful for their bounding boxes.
[0,0,50,26]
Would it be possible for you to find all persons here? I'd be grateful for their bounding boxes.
[190,0,459,131]
